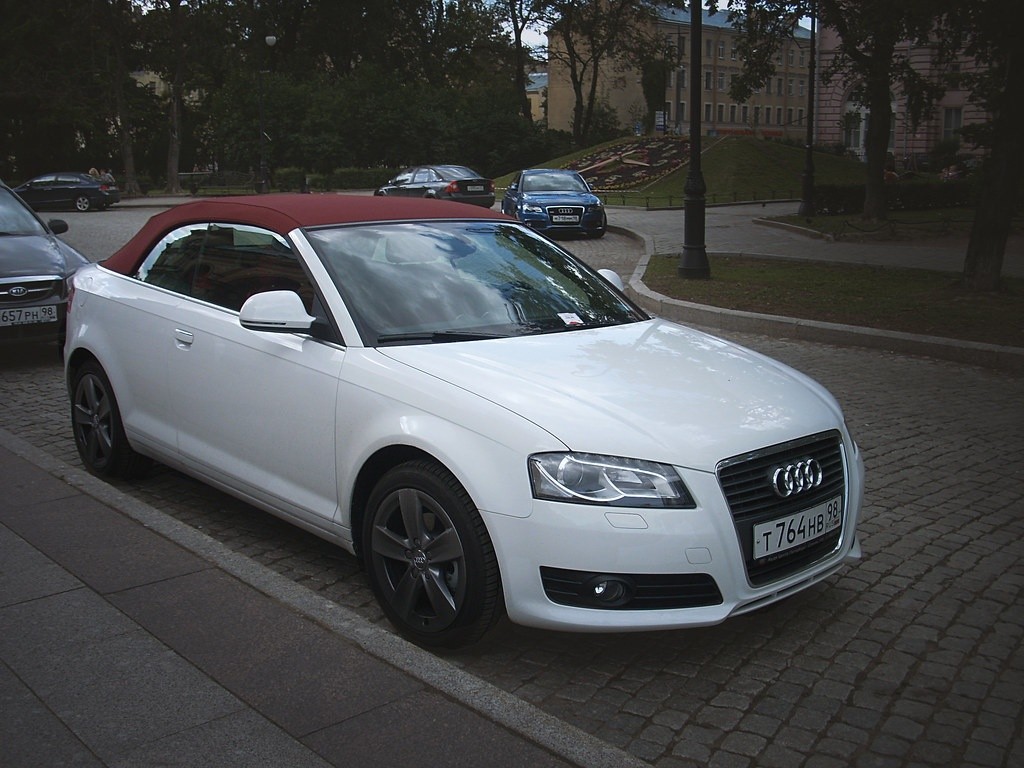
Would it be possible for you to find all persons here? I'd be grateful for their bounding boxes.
[90,168,116,182]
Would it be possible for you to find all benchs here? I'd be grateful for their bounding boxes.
[197,171,255,196]
[944,165,978,183]
[894,172,929,185]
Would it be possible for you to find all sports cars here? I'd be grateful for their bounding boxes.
[63,193,866,656]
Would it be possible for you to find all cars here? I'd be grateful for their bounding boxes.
[11,170,121,212]
[501,168,608,239]
[0,178,92,359]
[374,163,496,210]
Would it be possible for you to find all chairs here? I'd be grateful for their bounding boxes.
[386,235,438,264]
